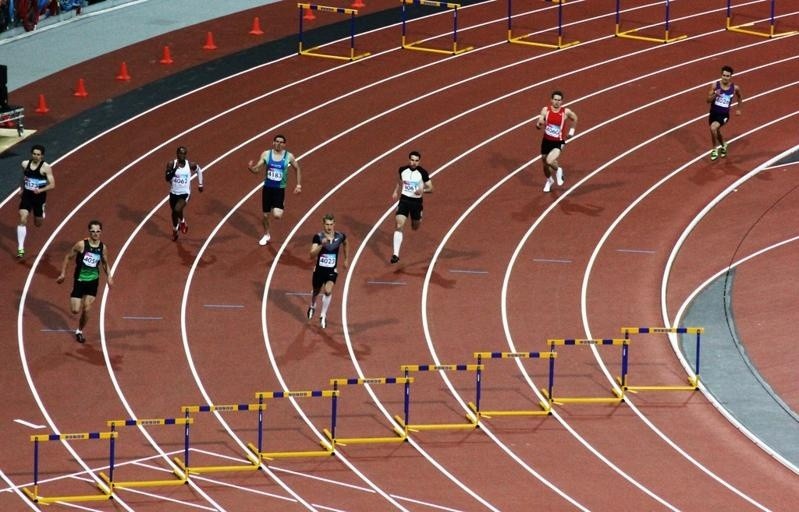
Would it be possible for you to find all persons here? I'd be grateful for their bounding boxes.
[247,134,304,246]
[55,219,116,344]
[534,90,580,194]
[705,65,744,162]
[164,146,205,241]
[389,151,434,265]
[14,144,57,262]
[307,213,350,329]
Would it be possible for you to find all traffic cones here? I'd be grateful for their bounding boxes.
[203,29,218,52]
[352,0,366,9]
[0,115,14,127]
[33,42,174,117]
[248,13,266,35]
[303,2,316,19]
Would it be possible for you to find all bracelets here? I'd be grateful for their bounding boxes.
[569,128,575,137]
[297,184,302,189]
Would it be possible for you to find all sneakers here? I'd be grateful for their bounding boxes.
[258,234,271,246]
[542,177,555,193]
[710,148,718,161]
[555,167,565,187]
[720,143,729,158]
[75,329,85,343]
[168,219,189,241]
[306,302,318,320]
[16,247,25,258]
[390,254,401,265]
[319,313,328,328]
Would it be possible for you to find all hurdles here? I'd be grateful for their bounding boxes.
[615,327,702,390]
[99,418,194,489]
[173,403,266,474]
[468,351,558,418]
[614,2,687,44]
[508,1,579,50]
[541,338,630,403]
[727,0,799,37]
[296,3,370,60]
[323,377,414,445]
[400,1,471,55]
[247,389,340,460]
[394,364,485,430]
[23,431,118,503]
